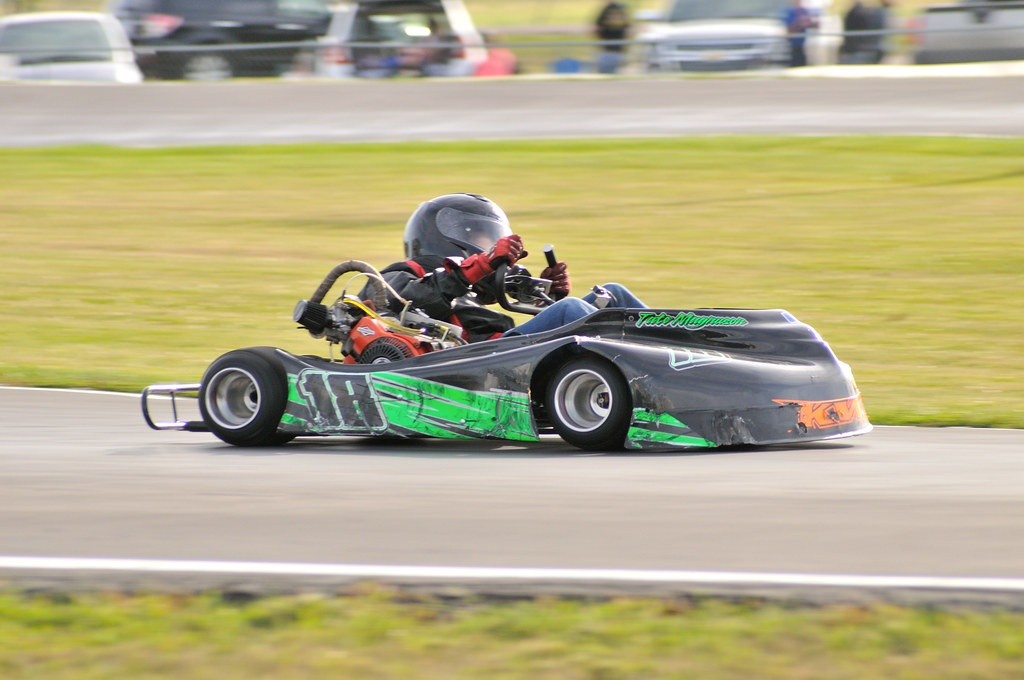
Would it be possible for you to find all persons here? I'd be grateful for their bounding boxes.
[342,193,648,367]
[595,2,627,74]
[784,1,817,68]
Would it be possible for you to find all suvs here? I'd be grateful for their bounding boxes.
[905,0,1024,70]
[631,0,845,71]
[108,0,336,84]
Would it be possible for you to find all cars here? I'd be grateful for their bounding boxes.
[316,0,505,90]
[0,11,144,86]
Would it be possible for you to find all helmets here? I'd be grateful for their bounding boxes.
[402,193,515,302]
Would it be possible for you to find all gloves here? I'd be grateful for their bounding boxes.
[540,263,570,300]
[477,233,524,275]
